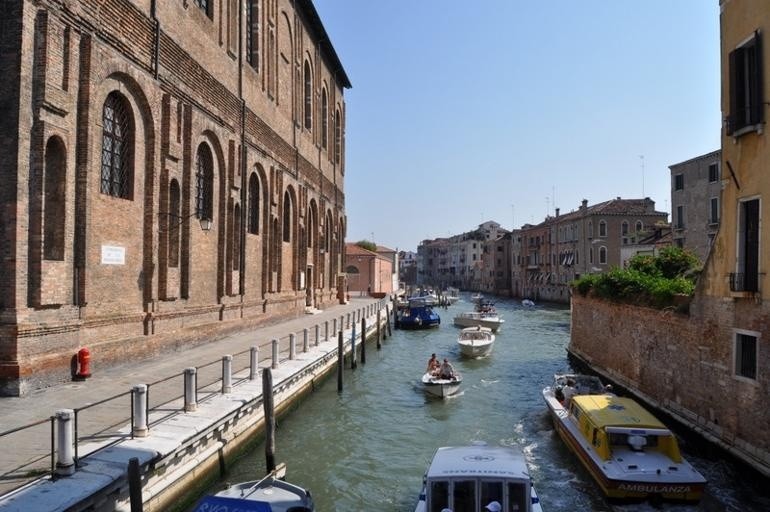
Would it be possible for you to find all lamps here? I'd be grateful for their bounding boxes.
[156,208,211,237]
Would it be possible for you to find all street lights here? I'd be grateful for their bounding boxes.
[357,258,362,290]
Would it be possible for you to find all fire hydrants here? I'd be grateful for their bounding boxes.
[77,347,92,381]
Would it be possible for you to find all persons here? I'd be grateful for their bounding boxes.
[604,384,618,397]
[480,306,494,316]
[561,379,577,408]
[439,358,454,379]
[425,353,441,376]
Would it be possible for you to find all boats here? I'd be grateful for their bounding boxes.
[540,372,713,504]
[522,299,537,310]
[451,298,504,335]
[470,292,484,303]
[454,325,497,361]
[191,474,318,512]
[394,284,460,331]
[419,367,464,400]
[408,443,545,512]
